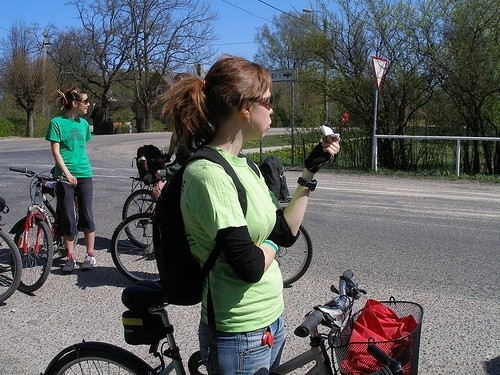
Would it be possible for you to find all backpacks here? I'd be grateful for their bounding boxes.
[259,154,290,200]
[152,147,247,306]
[136,144,168,185]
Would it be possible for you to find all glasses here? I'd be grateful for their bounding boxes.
[240,94,274,109]
[74,97,89,105]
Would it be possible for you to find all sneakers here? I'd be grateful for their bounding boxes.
[62,255,76,272]
[81,256,96,270]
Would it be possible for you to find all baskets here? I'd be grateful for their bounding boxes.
[334,301,424,375]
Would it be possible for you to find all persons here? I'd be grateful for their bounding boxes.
[46,86,96,272]
[148,126,191,201]
[157,55,341,375]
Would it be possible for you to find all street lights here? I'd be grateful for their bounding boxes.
[302,8,329,126]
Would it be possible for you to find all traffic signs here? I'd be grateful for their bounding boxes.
[269,68,298,82]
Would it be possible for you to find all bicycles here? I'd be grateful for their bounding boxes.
[111,199,314,290]
[42,279,425,374]
[121,176,167,253]
[7,167,83,295]
[0,197,23,303]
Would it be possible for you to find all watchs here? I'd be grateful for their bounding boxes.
[297,177,317,192]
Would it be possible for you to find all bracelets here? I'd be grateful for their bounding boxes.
[264,241,278,252]
